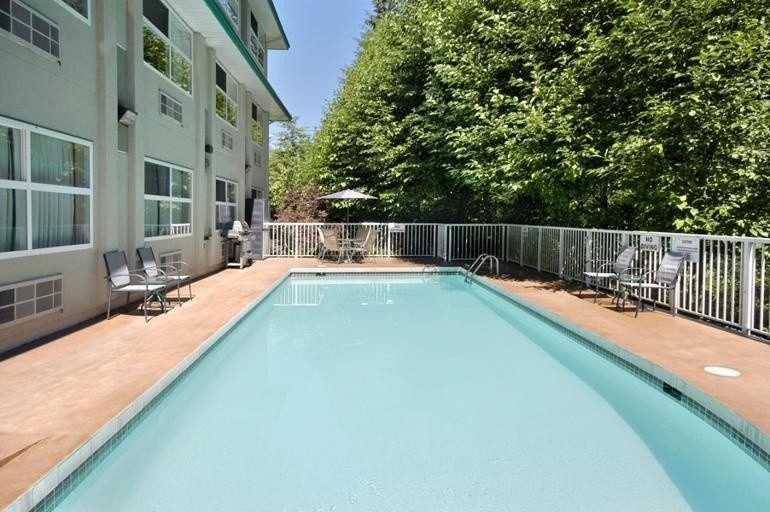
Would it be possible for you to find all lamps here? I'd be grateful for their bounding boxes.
[118,104,138,128]
[245,164,251,175]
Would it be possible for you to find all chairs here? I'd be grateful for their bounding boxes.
[618,251,688,318]
[579,246,636,304]
[317,227,371,264]
[103,250,168,323]
[136,247,193,308]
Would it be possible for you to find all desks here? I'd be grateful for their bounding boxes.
[221,230,254,269]
[136,278,174,313]
[609,275,646,312]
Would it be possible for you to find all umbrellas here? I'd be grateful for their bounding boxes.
[318,188,379,240]
